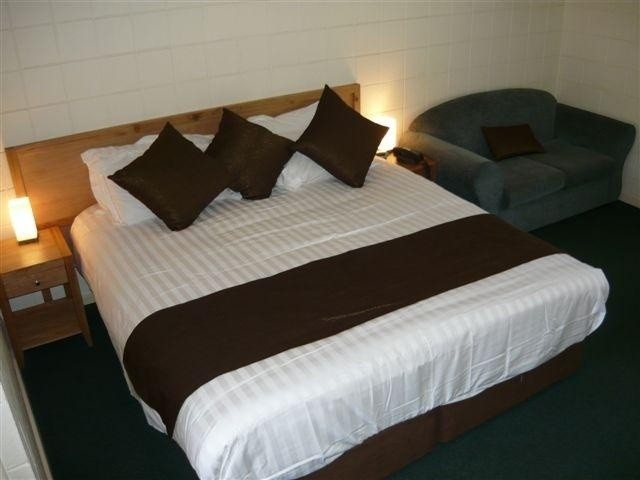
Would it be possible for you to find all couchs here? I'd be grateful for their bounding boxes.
[399,88,637,231]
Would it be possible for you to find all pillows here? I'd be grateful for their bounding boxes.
[204,107,304,199]
[294,84,389,188]
[481,124,545,161]
[80,134,240,225]
[107,121,231,232]
[244,100,338,189]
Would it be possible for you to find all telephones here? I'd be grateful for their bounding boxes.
[393,146,423,165]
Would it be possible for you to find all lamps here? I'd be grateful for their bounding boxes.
[373,115,397,153]
[5,198,39,244]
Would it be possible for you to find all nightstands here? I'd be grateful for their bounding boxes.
[2,227,98,368]
[382,151,438,187]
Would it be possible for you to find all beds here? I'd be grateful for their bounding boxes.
[4,84,610,480]
